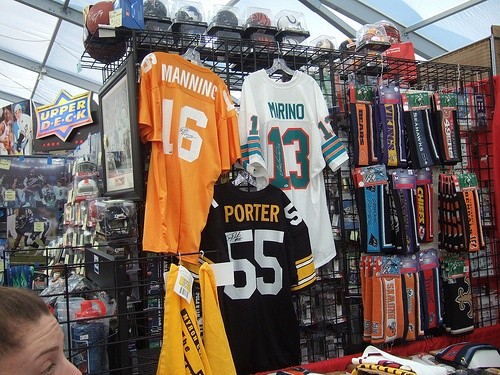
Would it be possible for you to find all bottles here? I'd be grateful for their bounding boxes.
[71,299,108,375]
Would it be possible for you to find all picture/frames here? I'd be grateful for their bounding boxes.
[97,51,142,200]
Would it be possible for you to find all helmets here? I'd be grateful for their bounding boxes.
[75,156,100,178]
[99,206,131,241]
[4,109,10,121]
[82,0,404,76]
[77,178,99,199]
[13,104,22,113]
[107,241,134,270]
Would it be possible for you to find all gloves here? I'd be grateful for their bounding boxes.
[16,133,24,152]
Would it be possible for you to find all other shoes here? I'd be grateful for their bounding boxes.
[16,240,55,257]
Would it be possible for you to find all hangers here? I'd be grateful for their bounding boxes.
[263,40,299,77]
[178,32,206,68]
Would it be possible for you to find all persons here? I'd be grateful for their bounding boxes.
[13,104,31,155]
[1,286,84,375]
[0,107,17,155]
[1,166,69,251]
